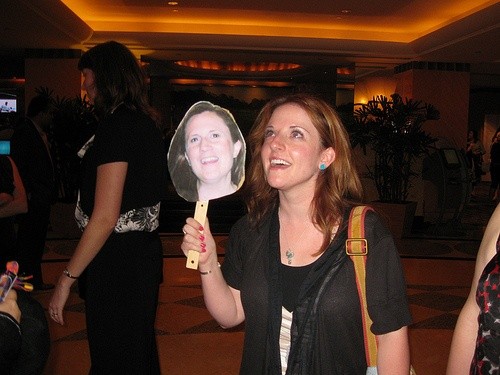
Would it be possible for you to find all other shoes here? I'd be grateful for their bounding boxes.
[34,283,55,291]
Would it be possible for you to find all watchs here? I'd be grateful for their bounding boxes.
[62,266,79,279]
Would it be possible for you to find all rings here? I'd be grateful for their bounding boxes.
[52,312,59,317]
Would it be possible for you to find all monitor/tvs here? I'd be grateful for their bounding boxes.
[442,147,461,168]
[0,99,17,113]
[0,139,11,155]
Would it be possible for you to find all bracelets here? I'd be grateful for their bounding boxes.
[198,262,222,275]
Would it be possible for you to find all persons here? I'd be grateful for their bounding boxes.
[166,100,248,202]
[0,154,35,280]
[445,200,500,375]
[464,130,500,204]
[27,90,61,207]
[0,288,49,375]
[48,40,169,375]
[180,93,415,375]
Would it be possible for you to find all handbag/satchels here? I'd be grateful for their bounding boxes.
[366,363,416,375]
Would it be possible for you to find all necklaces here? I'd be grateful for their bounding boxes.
[280,218,316,265]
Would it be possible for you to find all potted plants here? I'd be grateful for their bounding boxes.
[34,85,100,235]
[344,93,442,240]
